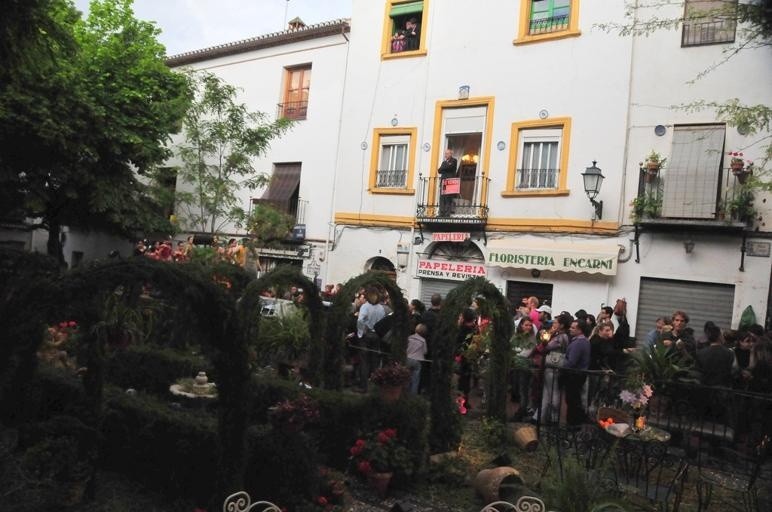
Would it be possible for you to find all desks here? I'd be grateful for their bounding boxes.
[606,422,673,492]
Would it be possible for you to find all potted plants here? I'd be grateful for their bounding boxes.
[648,148,667,171]
[718,200,729,221]
[644,157,668,183]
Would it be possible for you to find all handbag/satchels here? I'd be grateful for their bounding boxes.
[544,351,567,368]
[614,297,626,315]
[559,367,586,387]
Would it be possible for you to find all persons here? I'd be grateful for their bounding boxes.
[409,298,426,333]
[559,319,591,425]
[600,305,615,331]
[605,298,631,378]
[526,296,543,330]
[643,315,671,352]
[404,323,428,394]
[390,31,405,53]
[724,321,772,442]
[514,315,535,416]
[574,308,593,338]
[421,292,443,343]
[661,310,697,363]
[587,323,610,412]
[532,319,556,422]
[404,17,421,51]
[696,320,716,350]
[526,305,553,416]
[356,286,386,374]
[437,149,459,219]
[513,302,539,337]
[457,308,477,412]
[526,315,568,426]
[135,232,262,289]
[696,326,735,445]
[259,280,366,377]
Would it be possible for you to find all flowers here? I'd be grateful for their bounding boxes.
[727,152,744,162]
[617,369,656,416]
[746,160,754,168]
[349,425,409,472]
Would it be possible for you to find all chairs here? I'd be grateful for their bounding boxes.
[698,436,771,512]
[609,436,687,511]
[535,414,582,492]
[650,404,695,508]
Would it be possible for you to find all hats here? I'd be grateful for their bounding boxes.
[535,304,552,314]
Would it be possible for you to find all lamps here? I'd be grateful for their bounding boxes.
[684,236,694,253]
[582,160,605,220]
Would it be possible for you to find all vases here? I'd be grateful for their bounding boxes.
[516,426,543,453]
[746,166,750,170]
[474,462,527,510]
[731,162,744,170]
[635,416,646,433]
[363,469,394,497]
